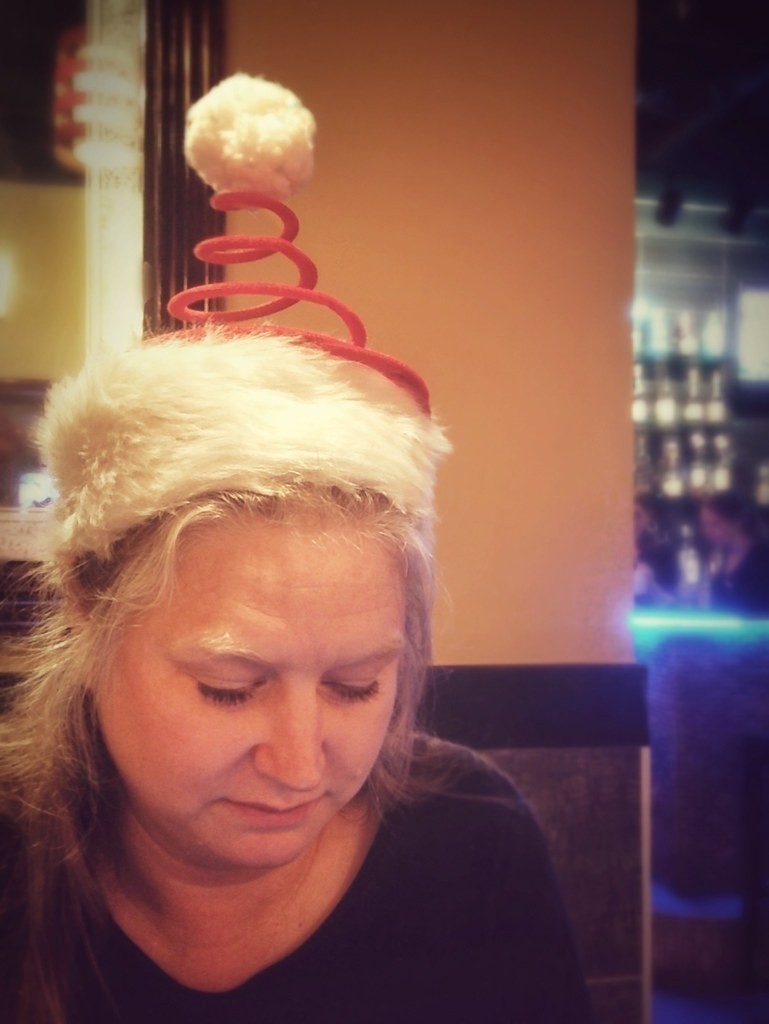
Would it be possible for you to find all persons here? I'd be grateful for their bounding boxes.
[631,486,769,623]
[0,73,588,1024]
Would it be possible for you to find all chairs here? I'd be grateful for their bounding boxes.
[425,662,652,1024]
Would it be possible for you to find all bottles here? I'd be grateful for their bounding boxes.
[632,363,726,428]
[673,523,702,606]
[634,432,730,499]
